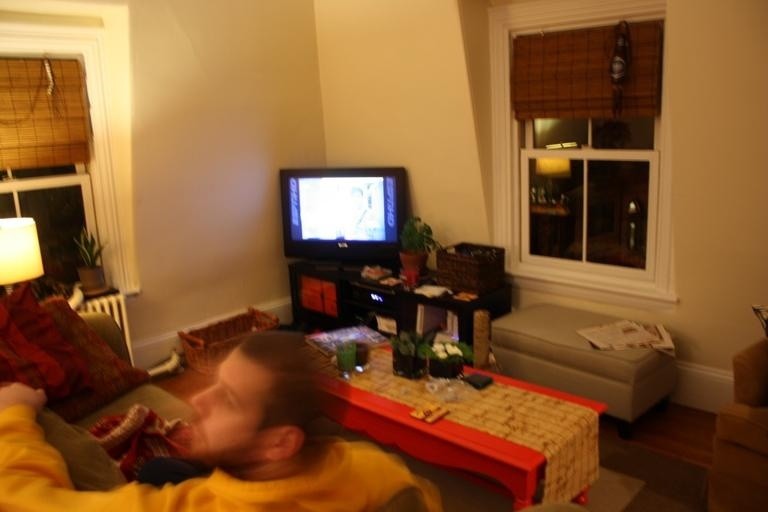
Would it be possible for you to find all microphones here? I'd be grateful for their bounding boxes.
[490,304,677,442]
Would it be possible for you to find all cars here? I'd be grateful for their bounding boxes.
[335,338,355,378]
[355,342,372,373]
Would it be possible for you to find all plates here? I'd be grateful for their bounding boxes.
[292,266,511,365]
[532,204,570,255]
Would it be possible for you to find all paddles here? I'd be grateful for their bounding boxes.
[304,416,708,512]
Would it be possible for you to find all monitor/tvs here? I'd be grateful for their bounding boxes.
[411,304,491,370]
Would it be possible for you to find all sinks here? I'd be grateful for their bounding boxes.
[0,287,431,512]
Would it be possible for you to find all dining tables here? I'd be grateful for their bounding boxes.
[390,330,429,379]
[429,333,472,377]
[399,216,438,275]
[71,226,105,291]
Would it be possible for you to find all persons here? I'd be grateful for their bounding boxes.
[0,330,444,512]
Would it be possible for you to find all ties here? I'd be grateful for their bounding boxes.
[277,163,412,272]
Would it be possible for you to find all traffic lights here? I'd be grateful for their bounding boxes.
[0,281,93,406]
[36,296,151,421]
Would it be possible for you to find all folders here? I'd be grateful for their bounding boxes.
[74,292,137,372]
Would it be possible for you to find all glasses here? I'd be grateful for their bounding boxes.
[0,216,45,294]
[536,157,571,208]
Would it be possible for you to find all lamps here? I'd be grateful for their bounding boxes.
[707,334,767,510]
